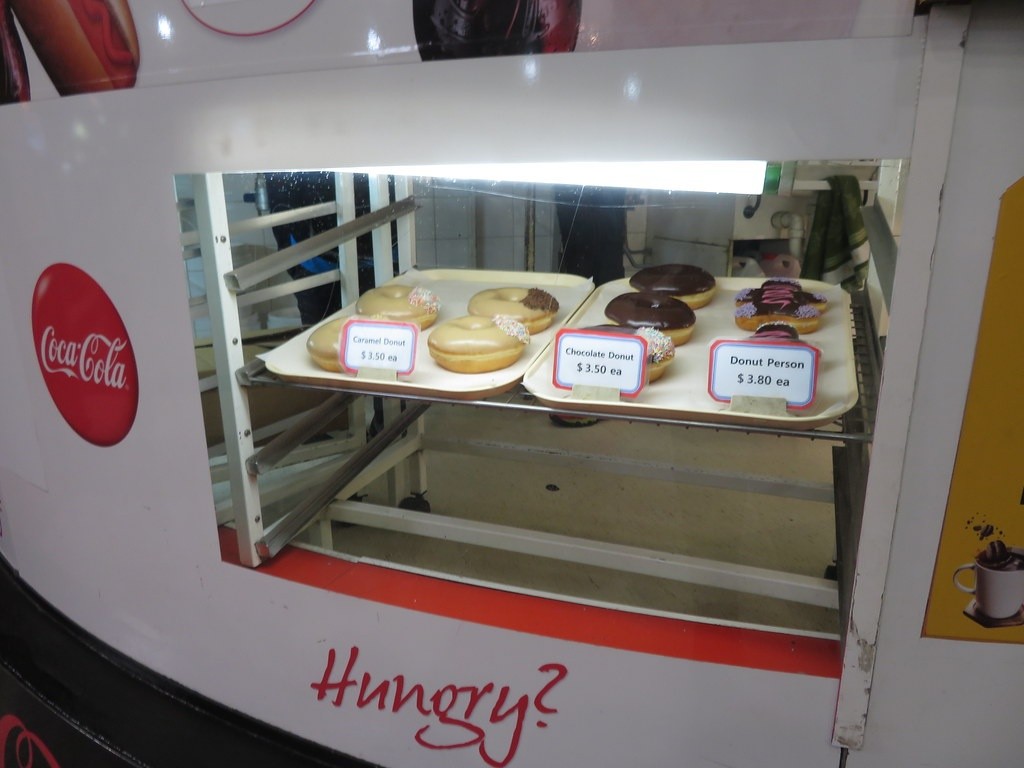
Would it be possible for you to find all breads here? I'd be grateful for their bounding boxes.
[745,321,804,342]
[735,277,827,335]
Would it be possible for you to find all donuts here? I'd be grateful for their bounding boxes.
[579,262,718,383]
[305,285,560,374]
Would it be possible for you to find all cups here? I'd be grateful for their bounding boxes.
[953,548,1024,619]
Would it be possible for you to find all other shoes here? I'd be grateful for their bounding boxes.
[370,416,408,441]
[548,414,602,426]
